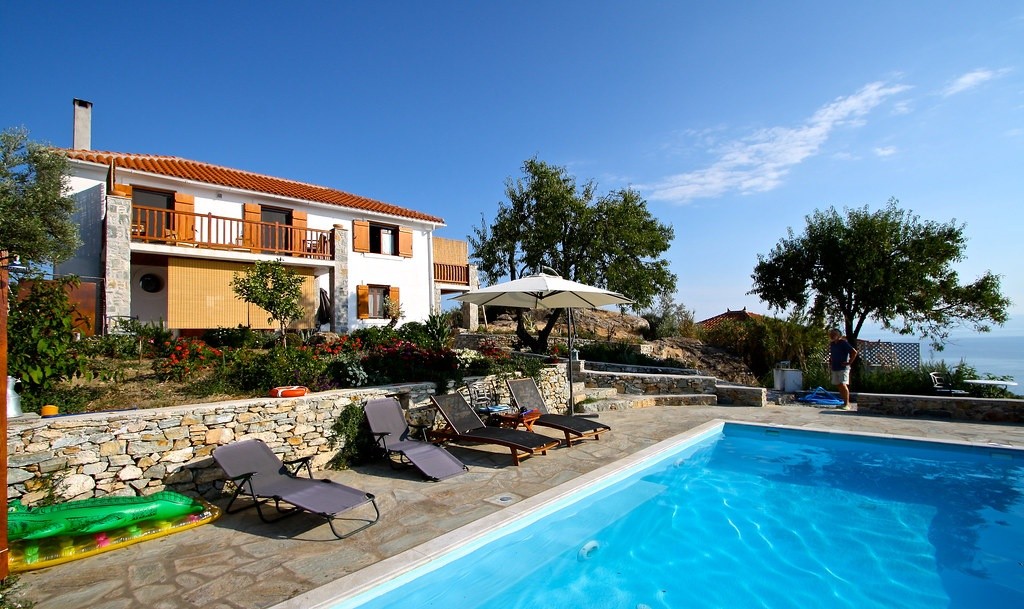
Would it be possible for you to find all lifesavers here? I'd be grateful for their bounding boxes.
[268,384,309,398]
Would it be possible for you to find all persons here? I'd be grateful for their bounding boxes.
[830,329,858,411]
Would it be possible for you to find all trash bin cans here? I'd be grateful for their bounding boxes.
[774,360,803,391]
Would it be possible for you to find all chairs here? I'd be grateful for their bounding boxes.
[303,234,326,260]
[363,395,470,484]
[928,371,970,397]
[421,391,563,467]
[505,376,612,448]
[165,229,198,248]
[210,437,382,540]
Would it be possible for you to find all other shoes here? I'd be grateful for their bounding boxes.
[836,405,851,410]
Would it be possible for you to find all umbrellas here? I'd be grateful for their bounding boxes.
[446,264,637,416]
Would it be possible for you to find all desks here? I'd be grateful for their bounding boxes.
[489,410,541,434]
[962,380,1018,398]
